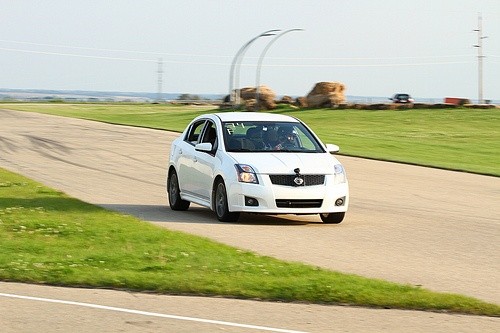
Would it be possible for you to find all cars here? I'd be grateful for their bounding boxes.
[168,112,349,224]
[388,93,411,103]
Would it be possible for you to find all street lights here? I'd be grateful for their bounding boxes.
[229,33,277,103]
[255,28,304,110]
[236,29,282,104]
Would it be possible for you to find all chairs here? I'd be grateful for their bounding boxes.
[222,127,243,150]
[260,129,283,150]
[245,127,265,150]
[203,126,217,149]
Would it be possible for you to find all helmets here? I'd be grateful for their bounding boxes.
[277,126,298,145]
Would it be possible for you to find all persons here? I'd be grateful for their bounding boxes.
[271,125,299,150]
